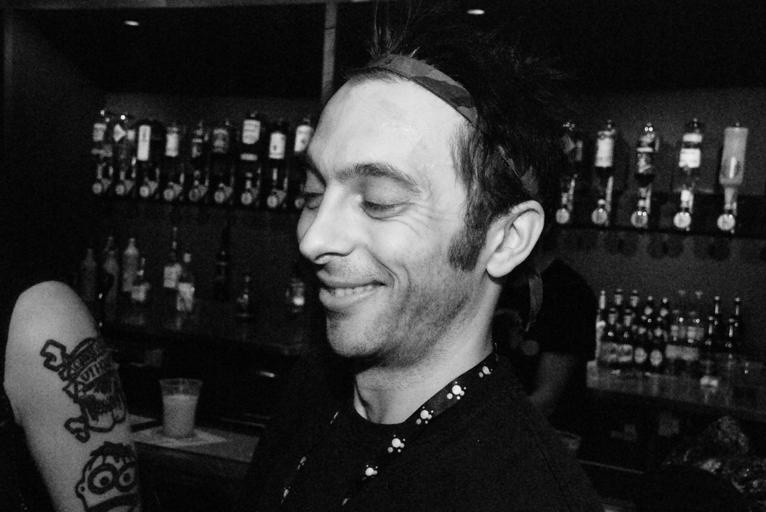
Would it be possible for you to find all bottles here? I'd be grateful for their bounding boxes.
[534,116,751,233]
[78,106,311,215]
[79,230,305,343]
[592,289,746,391]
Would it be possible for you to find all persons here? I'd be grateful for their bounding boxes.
[234,19,602,510]
[491,173,599,432]
[0,255,144,511]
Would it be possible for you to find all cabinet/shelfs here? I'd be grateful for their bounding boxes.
[90,113,766,512]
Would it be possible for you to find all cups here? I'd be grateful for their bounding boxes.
[158,378,203,437]
